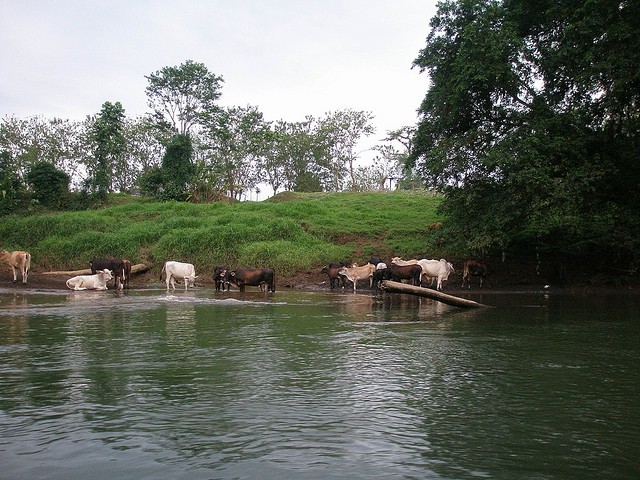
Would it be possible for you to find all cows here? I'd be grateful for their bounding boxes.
[122,259,131,287]
[420,259,454,290]
[65,268,114,291]
[160,260,199,290]
[329,263,351,268]
[368,258,388,289]
[224,268,276,292]
[220,269,230,291]
[462,259,484,289]
[339,262,376,291]
[387,262,422,286]
[213,266,224,291]
[88,259,123,290]
[0,249,34,284]
[390,256,419,266]
[320,266,350,292]
